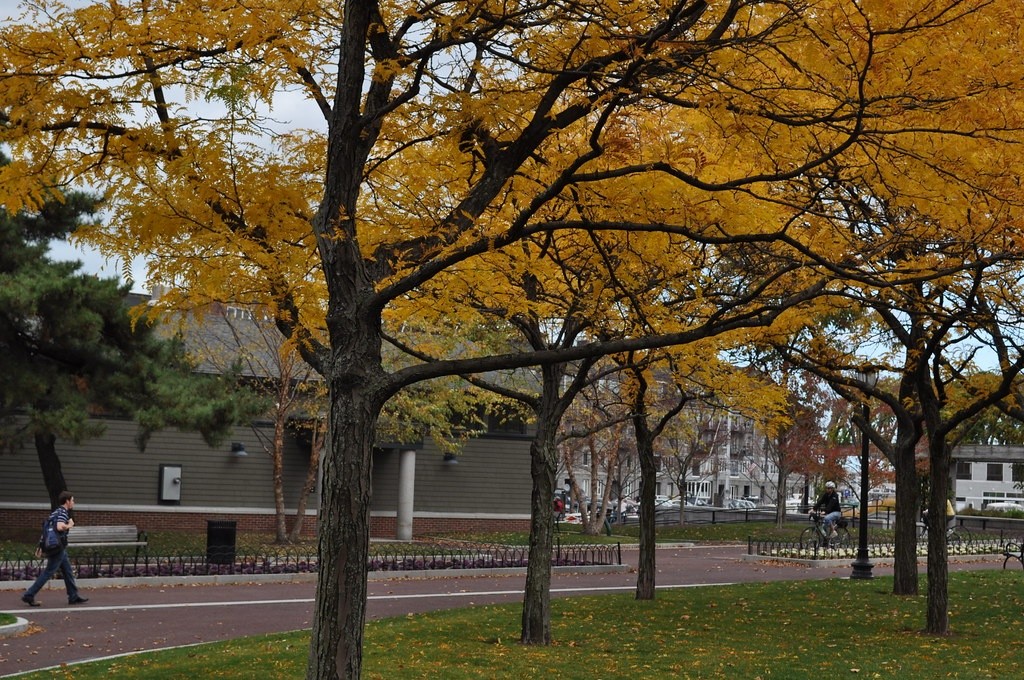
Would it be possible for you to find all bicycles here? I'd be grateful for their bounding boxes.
[800,508,851,551]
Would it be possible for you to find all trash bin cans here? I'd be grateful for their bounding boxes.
[206,520,237,564]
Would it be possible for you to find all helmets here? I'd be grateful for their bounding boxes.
[825,481,835,488]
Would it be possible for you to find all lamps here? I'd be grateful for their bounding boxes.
[231,441,248,457]
[444,451,460,465]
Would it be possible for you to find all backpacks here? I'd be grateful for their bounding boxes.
[40,511,69,555]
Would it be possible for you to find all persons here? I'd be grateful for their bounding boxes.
[553,496,563,513]
[634,497,641,515]
[607,498,627,521]
[808,481,841,550]
[923,498,955,537]
[21,492,89,606]
[844,489,850,500]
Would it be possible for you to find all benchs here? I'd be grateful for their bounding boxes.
[67,525,149,565]
[590,511,627,525]
[1003,542,1024,569]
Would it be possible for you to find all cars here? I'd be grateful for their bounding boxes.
[552,486,896,524]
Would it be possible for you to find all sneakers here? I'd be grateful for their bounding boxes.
[830,531,838,538]
[947,529,953,537]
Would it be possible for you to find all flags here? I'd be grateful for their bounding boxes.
[746,462,756,477]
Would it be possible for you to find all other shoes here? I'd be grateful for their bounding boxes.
[21,597,40,606]
[68,596,89,604]
